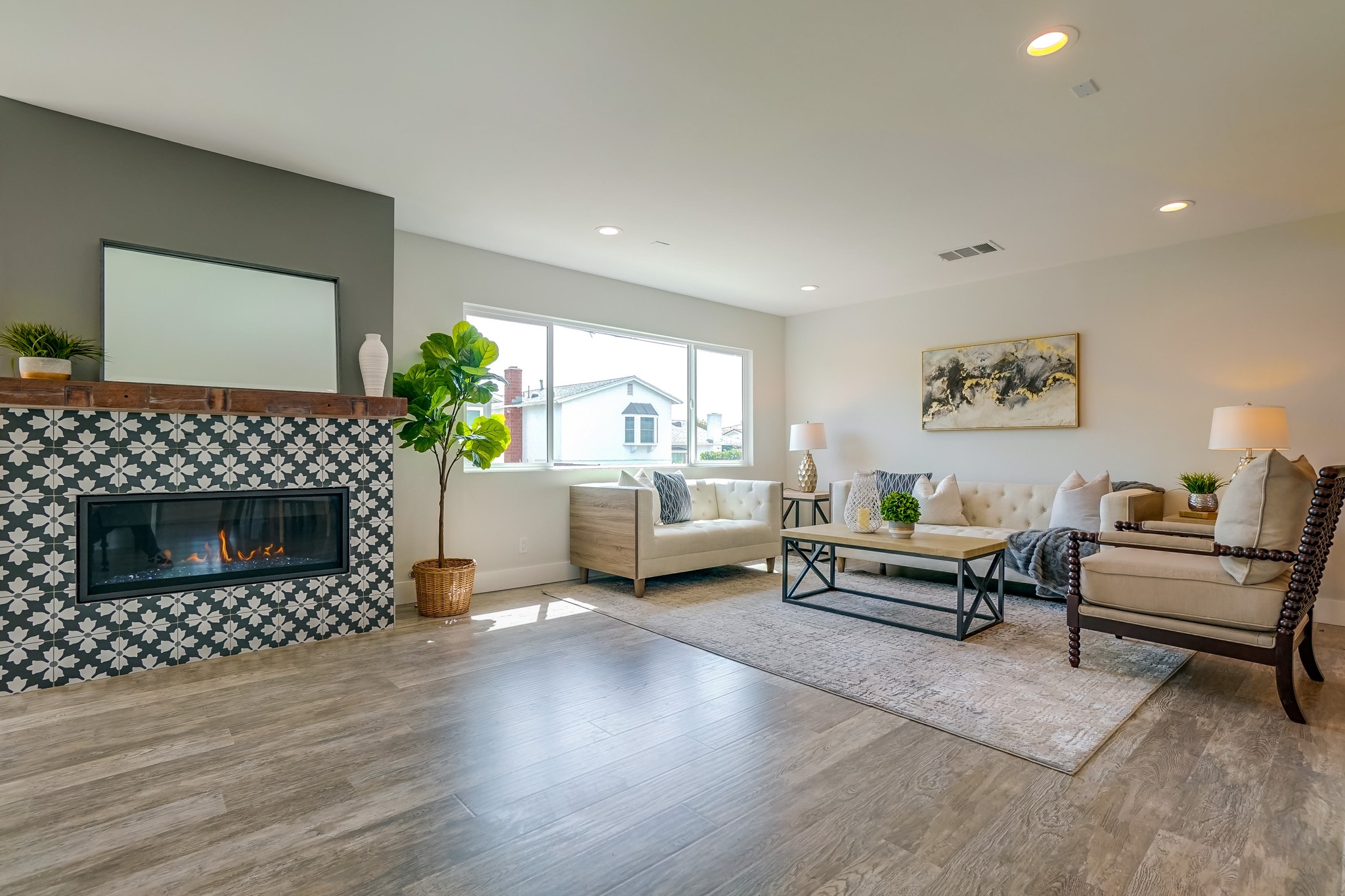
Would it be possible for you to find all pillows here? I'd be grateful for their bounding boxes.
[1049,470,1113,532]
[1214,449,1318,585]
[618,470,692,524]
[877,470,969,526]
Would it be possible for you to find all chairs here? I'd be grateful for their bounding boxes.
[1067,465,1345,725]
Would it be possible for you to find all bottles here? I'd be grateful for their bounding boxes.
[358,333,389,397]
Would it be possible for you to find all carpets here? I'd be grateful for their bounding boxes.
[542,555,1199,776]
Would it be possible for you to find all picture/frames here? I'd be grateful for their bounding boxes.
[922,332,1080,431]
[99,239,343,394]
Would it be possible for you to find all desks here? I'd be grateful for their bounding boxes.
[1163,514,1216,525]
[783,492,838,562]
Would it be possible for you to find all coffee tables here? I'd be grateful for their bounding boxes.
[780,523,1009,641]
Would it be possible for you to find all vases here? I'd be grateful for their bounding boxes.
[359,333,389,397]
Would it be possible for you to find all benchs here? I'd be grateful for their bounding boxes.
[570,478,784,598]
[833,480,1163,585]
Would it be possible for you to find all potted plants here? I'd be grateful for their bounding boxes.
[1169,471,1230,511]
[0,320,114,379]
[393,321,513,617]
[880,491,921,538]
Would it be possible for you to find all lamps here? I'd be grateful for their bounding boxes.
[1208,403,1291,481]
[789,421,827,492]
